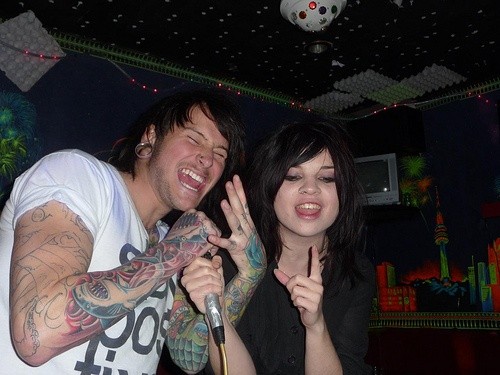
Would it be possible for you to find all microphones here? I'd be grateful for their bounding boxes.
[197,250,227,348]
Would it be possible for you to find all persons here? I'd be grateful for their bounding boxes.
[0,84,270,375]
[179,115,379,375]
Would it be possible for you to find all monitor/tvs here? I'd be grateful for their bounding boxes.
[354,153,400,208]
